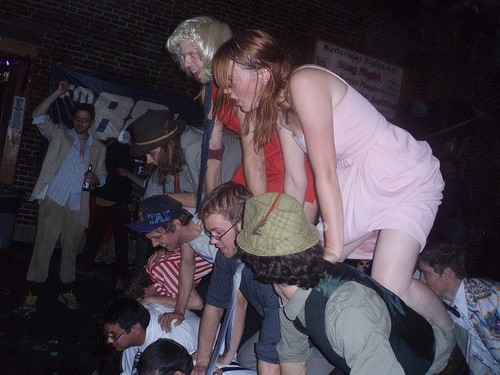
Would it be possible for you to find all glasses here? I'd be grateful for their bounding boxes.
[204,222,237,241]
[104,329,127,341]
[73,116,89,122]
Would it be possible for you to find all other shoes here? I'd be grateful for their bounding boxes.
[57,291,82,311]
[20,294,38,318]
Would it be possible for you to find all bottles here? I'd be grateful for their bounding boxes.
[82,164,93,191]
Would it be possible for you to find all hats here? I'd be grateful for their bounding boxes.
[124,196,183,232]
[124,109,187,156]
[234,193,321,256]
